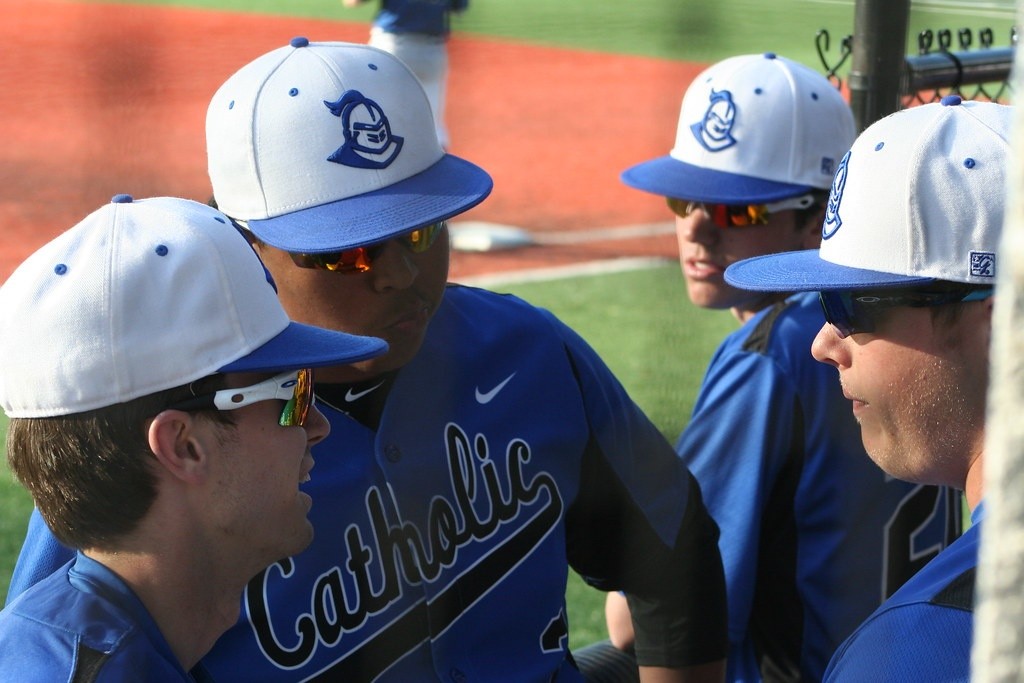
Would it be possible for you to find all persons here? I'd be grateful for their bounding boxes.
[0,194,389,683]
[724,95,1008,683]
[346,0,469,146]
[606,52,961,683]
[4,37,730,683]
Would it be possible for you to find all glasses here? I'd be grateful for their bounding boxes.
[665,195,815,229]
[817,286,994,340]
[287,221,445,273]
[176,367,315,428]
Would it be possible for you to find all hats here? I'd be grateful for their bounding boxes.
[0,192,390,420]
[724,96,1005,292]
[204,36,493,254]
[619,52,856,207]
